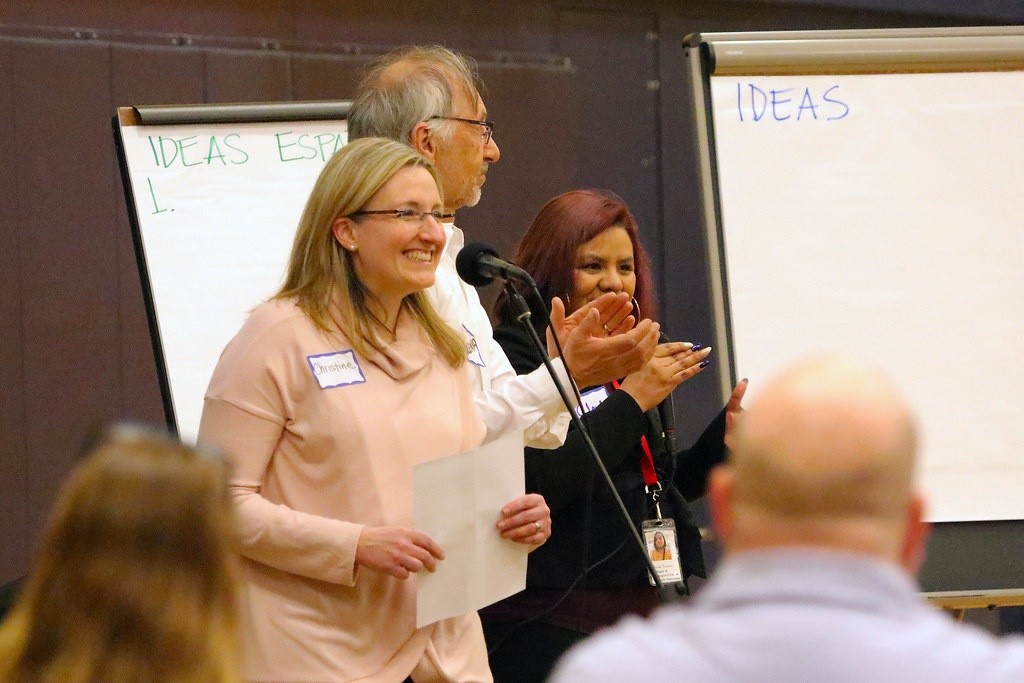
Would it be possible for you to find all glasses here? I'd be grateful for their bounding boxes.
[408,116,495,145]
[342,206,454,230]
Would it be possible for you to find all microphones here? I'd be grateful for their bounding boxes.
[656,330,678,457]
[456,242,536,288]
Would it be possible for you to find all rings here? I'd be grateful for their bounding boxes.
[535,523,541,533]
[604,325,612,331]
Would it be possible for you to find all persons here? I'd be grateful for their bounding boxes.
[650,531,671,561]
[193,137,551,683]
[475,190,748,683]
[0,417,251,683]
[551,356,1024,683]
[345,43,659,452]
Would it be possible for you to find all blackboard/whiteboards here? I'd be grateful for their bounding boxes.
[681,25,1024,610]
[111,101,358,446]
